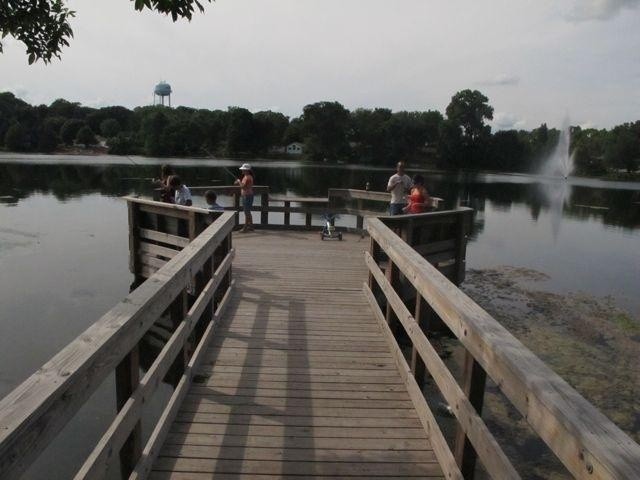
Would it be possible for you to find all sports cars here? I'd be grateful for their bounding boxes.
[319,212,343,241]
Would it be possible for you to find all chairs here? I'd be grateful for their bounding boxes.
[246,225,255,232]
[238,225,248,233]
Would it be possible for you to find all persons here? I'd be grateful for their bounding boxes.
[387,161,412,215]
[153,164,179,203]
[204,190,220,210]
[234,163,255,232]
[169,174,192,239]
[402,174,430,214]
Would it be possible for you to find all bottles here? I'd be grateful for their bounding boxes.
[239,163,252,171]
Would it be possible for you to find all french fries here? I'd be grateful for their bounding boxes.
[102,134,159,183]
[189,142,240,184]
